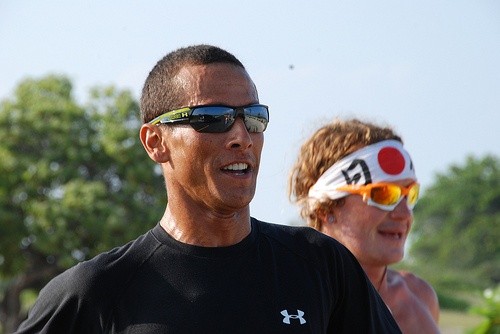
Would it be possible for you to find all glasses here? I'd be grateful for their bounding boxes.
[147,103,269,134]
[336,182,421,210]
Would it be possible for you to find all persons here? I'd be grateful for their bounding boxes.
[286,118,440,334]
[14,43,407,334]
